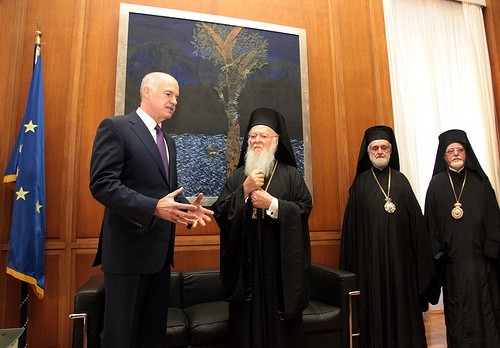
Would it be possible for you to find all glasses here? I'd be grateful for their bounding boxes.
[247,134,279,140]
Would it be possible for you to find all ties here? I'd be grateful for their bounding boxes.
[154,124,169,183]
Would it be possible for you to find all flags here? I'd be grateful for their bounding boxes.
[2,48,46,300]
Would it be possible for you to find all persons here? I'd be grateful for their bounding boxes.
[88,71,215,348]
[338,125,429,348]
[211,107,313,348]
[423,129,500,348]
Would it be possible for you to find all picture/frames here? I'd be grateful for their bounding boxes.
[114,3,315,208]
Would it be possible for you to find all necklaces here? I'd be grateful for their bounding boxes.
[371,167,396,214]
[447,169,468,219]
[252,160,279,218]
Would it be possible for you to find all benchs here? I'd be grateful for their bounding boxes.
[69,263,360,347]
